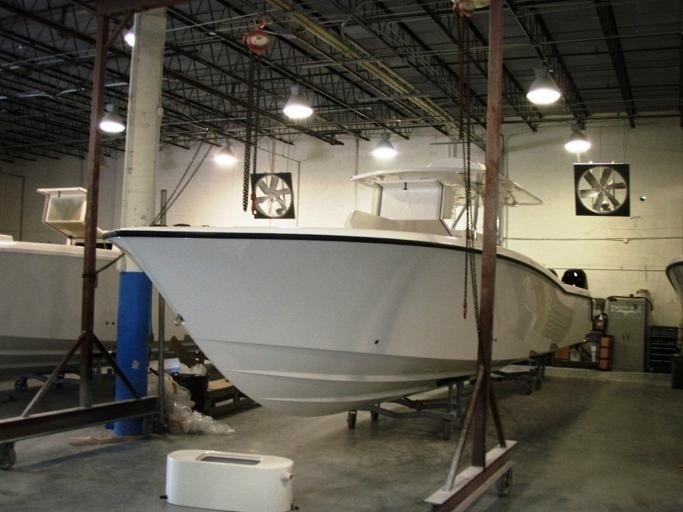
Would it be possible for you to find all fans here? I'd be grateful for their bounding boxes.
[574,163,629,218]
[249,172,294,219]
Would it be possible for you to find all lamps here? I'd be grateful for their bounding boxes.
[368,132,398,161]
[563,123,592,154]
[283,84,314,121]
[98,102,127,135]
[526,64,562,106]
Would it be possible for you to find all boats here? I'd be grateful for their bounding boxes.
[0,236,191,375]
[96,223,595,419]
